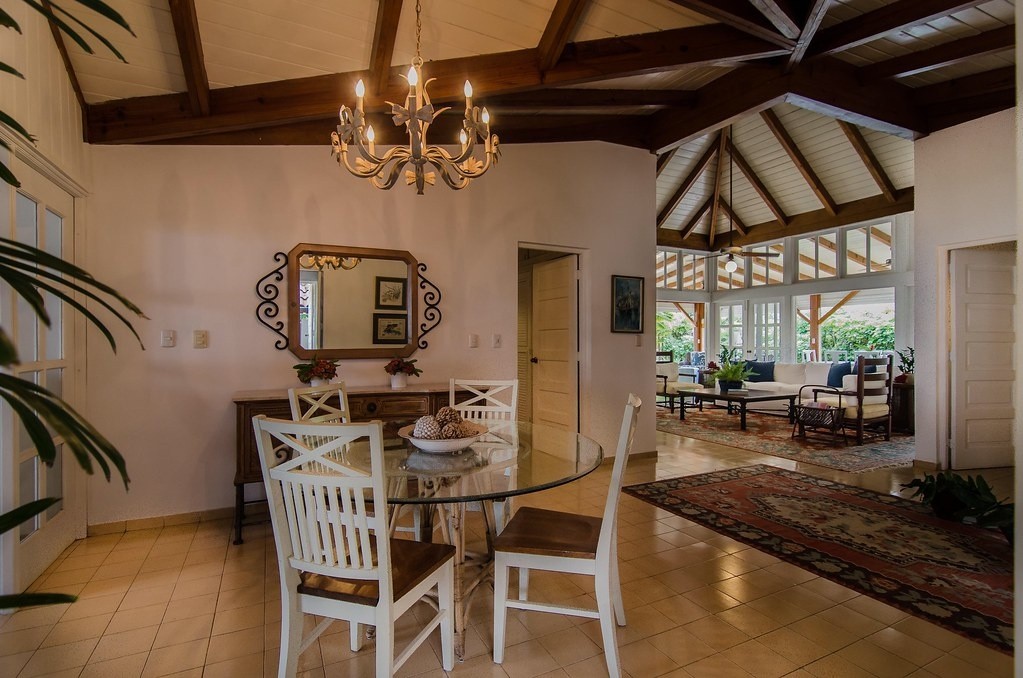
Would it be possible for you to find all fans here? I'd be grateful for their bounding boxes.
[688,123,780,261]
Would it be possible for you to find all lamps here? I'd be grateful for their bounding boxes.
[330,0,502,197]
[725,254,738,273]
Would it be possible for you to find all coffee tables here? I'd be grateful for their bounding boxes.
[676,387,799,431]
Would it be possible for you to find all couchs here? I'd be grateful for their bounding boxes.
[714,359,894,415]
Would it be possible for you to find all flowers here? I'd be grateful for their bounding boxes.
[293,354,341,384]
[384,353,424,377]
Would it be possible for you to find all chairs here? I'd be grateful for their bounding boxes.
[252,378,643,678]
[655,350,703,414]
[812,355,894,446]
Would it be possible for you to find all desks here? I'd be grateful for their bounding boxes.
[286,418,604,663]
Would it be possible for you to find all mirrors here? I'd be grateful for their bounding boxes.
[255,243,442,361]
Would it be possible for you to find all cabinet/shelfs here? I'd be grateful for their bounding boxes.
[231,382,502,546]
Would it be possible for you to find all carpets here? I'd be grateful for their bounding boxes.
[621,404,1015,656]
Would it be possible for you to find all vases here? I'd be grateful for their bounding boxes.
[310,376,329,387]
[390,372,408,388]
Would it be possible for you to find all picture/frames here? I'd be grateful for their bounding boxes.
[610,274,646,334]
[373,276,408,345]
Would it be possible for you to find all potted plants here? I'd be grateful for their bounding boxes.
[716,344,760,392]
[894,346,914,387]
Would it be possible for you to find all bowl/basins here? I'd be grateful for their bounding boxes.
[397,448,488,477]
[397,421,489,453]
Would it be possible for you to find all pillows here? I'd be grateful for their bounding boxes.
[729,359,775,382]
[803,356,878,388]
